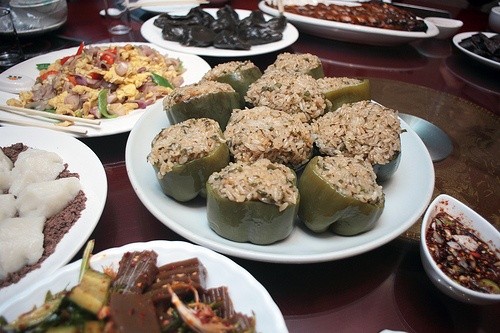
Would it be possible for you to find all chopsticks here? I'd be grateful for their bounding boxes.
[0,102,100,134]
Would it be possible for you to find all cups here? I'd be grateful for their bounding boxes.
[104,0,131,35]
[0,7,25,67]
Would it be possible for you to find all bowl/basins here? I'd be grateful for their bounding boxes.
[425,17,464,39]
[422,195,500,304]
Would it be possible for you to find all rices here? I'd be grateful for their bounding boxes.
[147,51,401,210]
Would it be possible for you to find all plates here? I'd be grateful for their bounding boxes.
[140,7,299,57]
[453,31,500,70]
[136,1,200,13]
[0,42,212,136]
[0,240,289,333]
[124,79,436,264]
[0,126,107,308]
[258,0,439,44]
